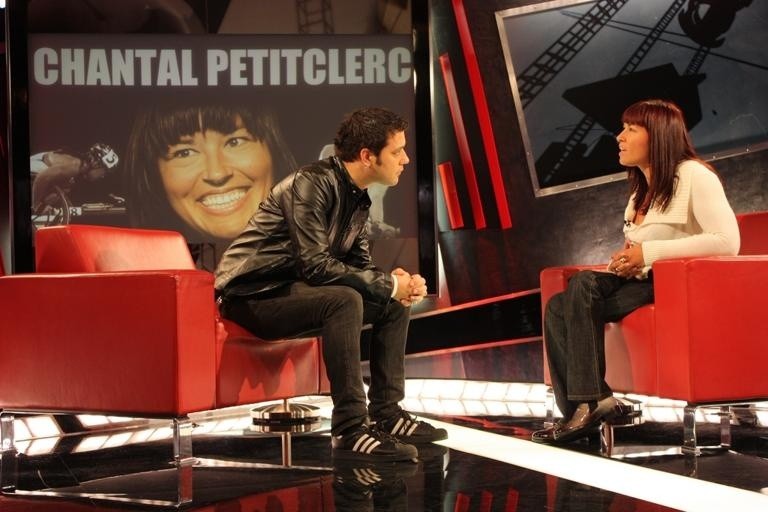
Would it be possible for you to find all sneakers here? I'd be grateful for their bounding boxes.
[372,405,448,443]
[330,423,419,463]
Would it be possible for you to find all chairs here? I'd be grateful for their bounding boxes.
[538,208,768,454]
[0,226,334,509]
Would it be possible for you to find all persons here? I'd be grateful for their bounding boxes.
[329,442,449,512]
[120,101,300,276]
[213,107,447,463]
[30,143,119,216]
[532,98,742,442]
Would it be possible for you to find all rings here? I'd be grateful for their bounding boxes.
[619,257,628,264]
[614,267,621,273]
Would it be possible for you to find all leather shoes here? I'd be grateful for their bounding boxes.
[532,420,603,442]
[554,400,623,441]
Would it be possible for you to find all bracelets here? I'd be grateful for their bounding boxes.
[390,274,398,298]
